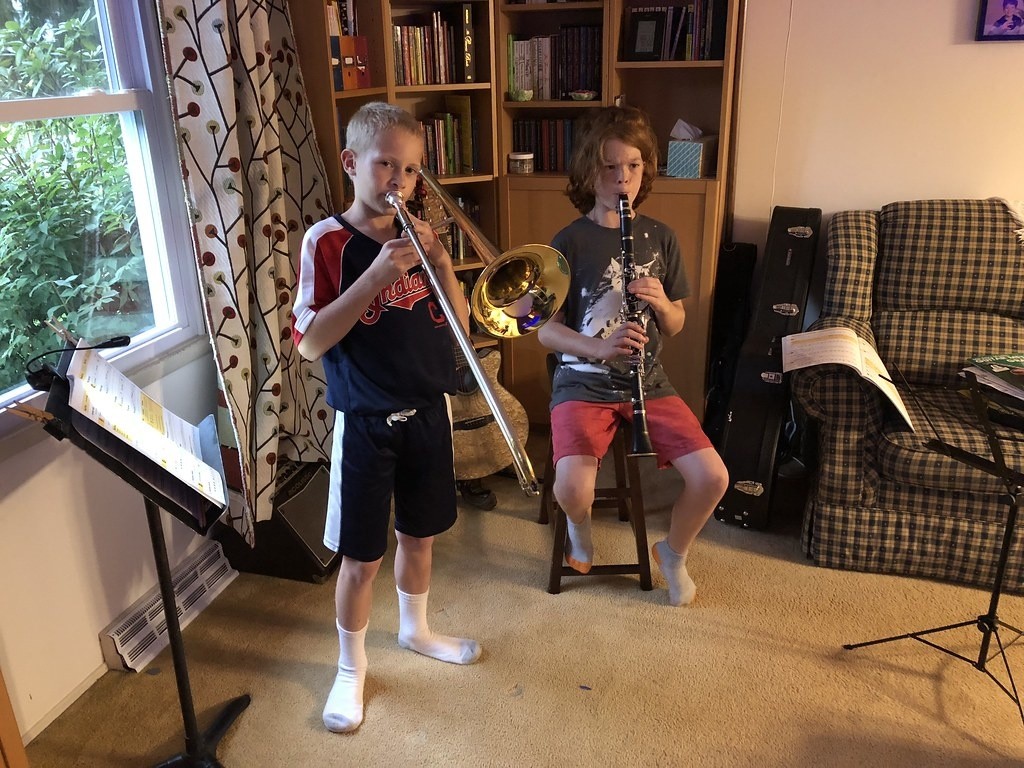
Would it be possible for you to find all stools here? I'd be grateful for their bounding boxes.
[537,352,653,594]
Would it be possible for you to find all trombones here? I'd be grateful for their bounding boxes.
[385,164,572,498]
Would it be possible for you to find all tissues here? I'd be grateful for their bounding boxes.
[665,117,719,179]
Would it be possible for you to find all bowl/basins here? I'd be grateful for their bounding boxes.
[509,89,534,101]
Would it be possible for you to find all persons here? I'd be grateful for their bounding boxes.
[288,100,484,733]
[539,105,730,606]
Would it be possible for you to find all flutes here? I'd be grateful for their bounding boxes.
[617,193,660,457]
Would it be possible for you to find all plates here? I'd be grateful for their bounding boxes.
[571,90,598,101]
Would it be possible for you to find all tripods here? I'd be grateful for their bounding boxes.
[841,486,1024,725]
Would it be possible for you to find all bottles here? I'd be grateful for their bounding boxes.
[508,152,534,175]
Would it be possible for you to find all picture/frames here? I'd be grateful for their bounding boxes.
[975,0,1024,41]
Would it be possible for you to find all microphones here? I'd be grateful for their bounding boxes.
[25,336,132,391]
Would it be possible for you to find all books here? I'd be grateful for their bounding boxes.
[327,1,716,333]
[782,327,917,432]
[956,352,1024,432]
[66,337,227,509]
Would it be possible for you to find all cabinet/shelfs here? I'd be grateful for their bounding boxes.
[288,1,746,432]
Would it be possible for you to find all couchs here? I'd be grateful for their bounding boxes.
[790,198,1024,597]
